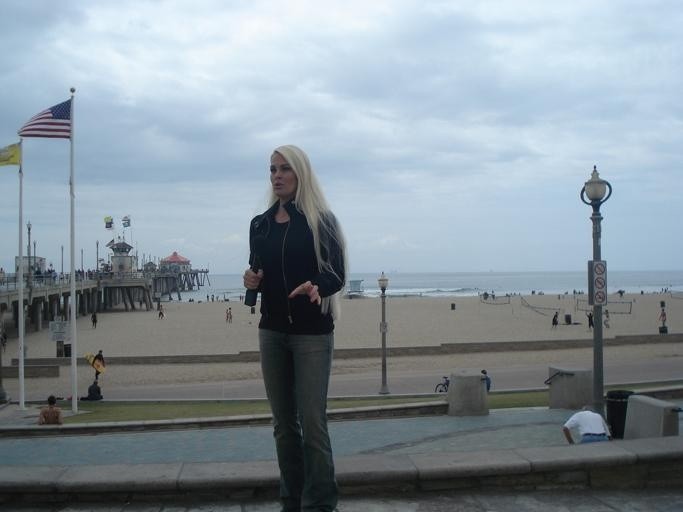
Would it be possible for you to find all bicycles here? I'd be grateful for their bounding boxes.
[435,376,450,392]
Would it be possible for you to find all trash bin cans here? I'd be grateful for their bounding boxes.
[57,341,71,357]
[565,315,571,324]
[607,391,635,438]
[452,304,456,310]
[659,327,667,336]
[252,307,255,314]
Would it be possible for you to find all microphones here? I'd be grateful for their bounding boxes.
[243,233,266,306]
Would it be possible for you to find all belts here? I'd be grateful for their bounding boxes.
[582,432,607,436]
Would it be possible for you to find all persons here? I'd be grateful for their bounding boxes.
[38,395,62,424]
[87,381,103,399]
[157,304,164,319]
[90,312,96,329]
[552,311,558,328]
[0,264,56,287]
[482,287,669,301]
[563,404,612,444]
[480,369,490,392]
[658,309,666,327]
[242,144,350,512]
[91,349,104,380]
[0,329,8,352]
[186,293,244,324]
[585,311,594,330]
[602,309,611,328]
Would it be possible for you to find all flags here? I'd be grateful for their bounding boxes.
[121,215,130,228]
[16,98,71,139]
[105,239,113,247]
[0,141,21,168]
[103,216,113,229]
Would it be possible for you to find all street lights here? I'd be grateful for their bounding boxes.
[378,271,391,394]
[26,220,99,289]
[580,164,612,420]
